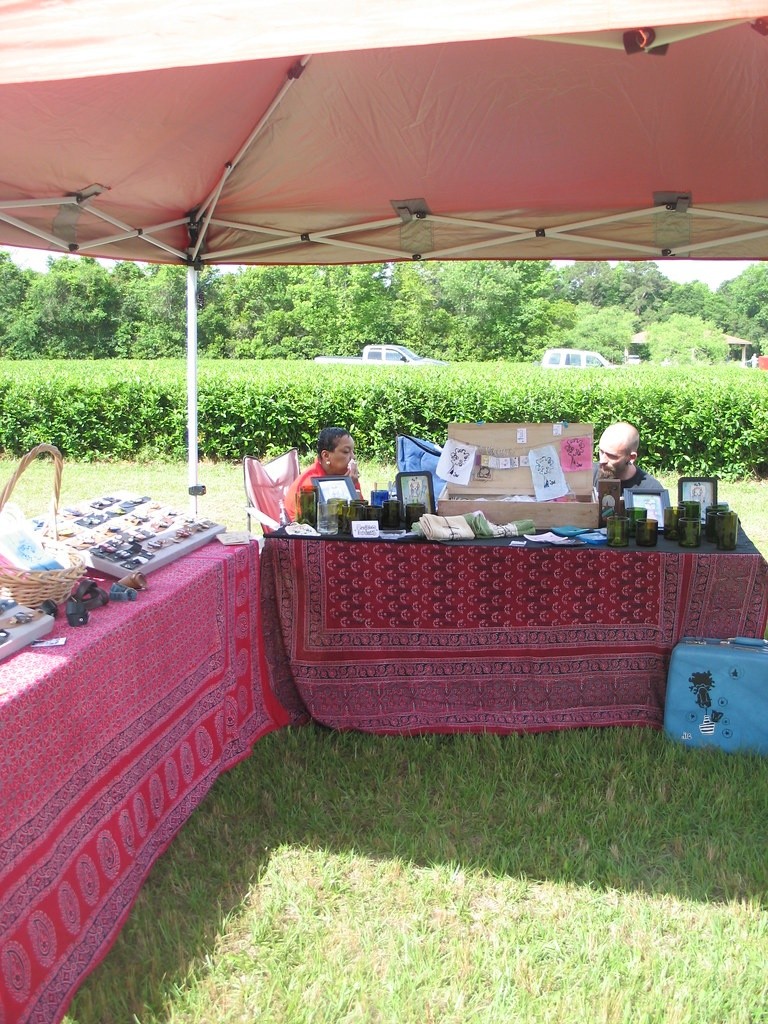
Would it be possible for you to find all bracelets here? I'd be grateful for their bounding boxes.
[356,490,361,492]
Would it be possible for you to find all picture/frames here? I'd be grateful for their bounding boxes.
[311,475,361,506]
[677,476,717,528]
[397,471,436,523]
[623,488,671,534]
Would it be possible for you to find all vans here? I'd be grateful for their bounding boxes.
[539,347,611,368]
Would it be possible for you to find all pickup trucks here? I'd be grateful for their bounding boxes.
[313,342,444,367]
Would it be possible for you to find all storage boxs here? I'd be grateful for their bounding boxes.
[438,422,599,529]
[598,479,621,526]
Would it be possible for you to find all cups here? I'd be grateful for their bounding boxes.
[364,505,384,531]
[383,500,402,530]
[328,498,348,529]
[294,484,319,527]
[606,516,629,547]
[406,503,425,534]
[678,501,702,535]
[350,499,368,527]
[635,518,659,546]
[342,503,363,534]
[663,506,685,540]
[625,507,647,538]
[679,517,701,548]
[317,501,338,536]
[372,489,389,505]
[705,505,739,551]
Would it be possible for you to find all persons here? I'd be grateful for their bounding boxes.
[750,353,757,368]
[593,423,663,499]
[282,428,364,522]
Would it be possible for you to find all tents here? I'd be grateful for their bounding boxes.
[0,0,768,520]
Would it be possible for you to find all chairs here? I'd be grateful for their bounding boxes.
[387,432,446,511]
[242,447,302,534]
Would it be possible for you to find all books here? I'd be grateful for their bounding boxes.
[216,531,250,545]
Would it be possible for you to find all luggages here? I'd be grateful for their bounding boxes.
[663,636,768,760]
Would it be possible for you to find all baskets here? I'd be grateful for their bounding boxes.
[0,442,88,608]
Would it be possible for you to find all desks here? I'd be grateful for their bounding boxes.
[259,518,768,737]
[0,537,260,1024]
